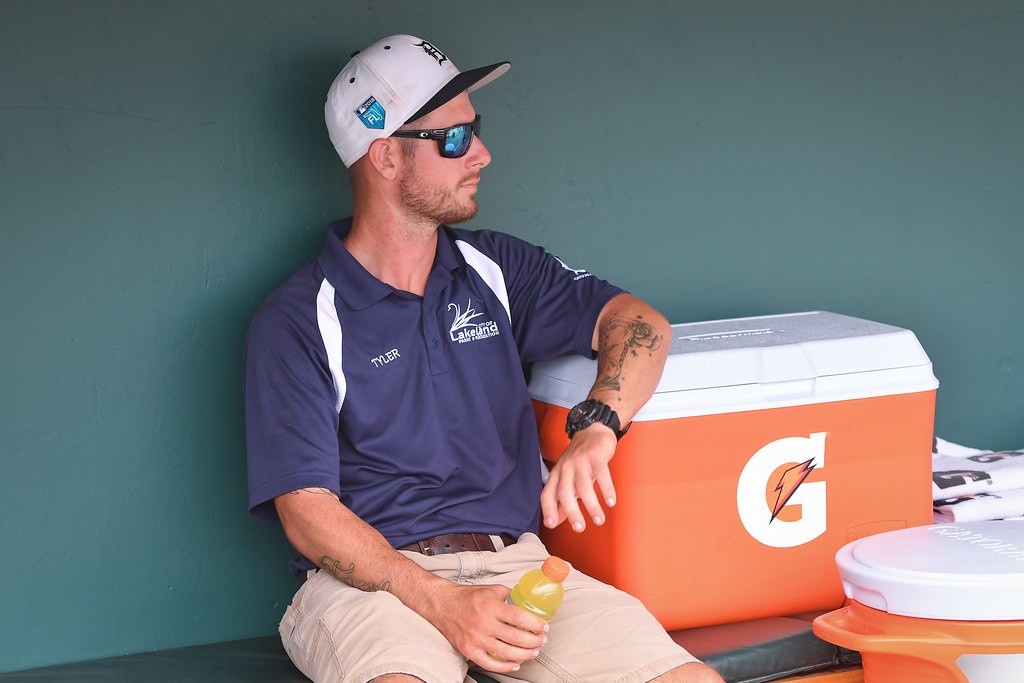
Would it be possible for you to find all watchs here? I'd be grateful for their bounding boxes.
[564,399,634,443]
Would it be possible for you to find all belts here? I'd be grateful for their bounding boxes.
[395,535,518,557]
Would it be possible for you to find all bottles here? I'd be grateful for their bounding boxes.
[483,555,570,664]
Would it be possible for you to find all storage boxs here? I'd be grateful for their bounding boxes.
[526,311,939,632]
[812,522,1024,683]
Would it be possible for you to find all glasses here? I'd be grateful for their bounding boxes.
[388,114,482,159]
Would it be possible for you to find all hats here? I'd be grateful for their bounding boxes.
[325,34,511,168]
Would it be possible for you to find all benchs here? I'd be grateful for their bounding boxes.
[0,616,838,683]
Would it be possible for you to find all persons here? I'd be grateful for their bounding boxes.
[242,32,734,682]
